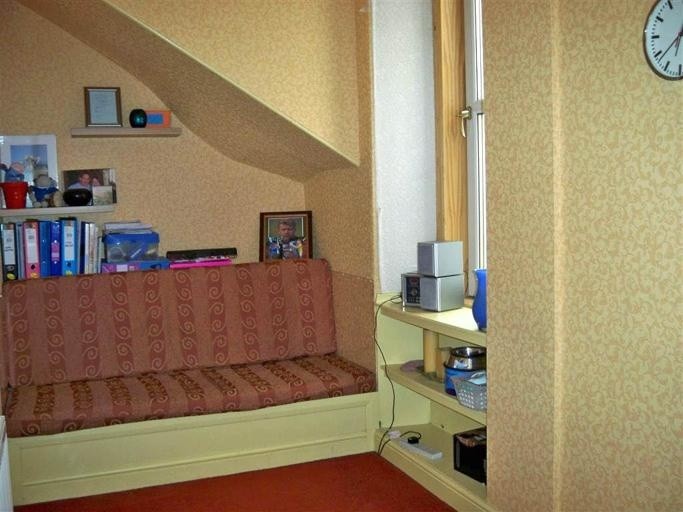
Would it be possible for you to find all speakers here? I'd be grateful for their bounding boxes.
[418,275,466,312]
[401,266,424,308]
[417,240,466,277]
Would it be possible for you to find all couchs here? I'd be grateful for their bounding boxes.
[1,260,377,507]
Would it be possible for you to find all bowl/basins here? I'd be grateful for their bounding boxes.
[441,363,486,397]
[446,347,486,370]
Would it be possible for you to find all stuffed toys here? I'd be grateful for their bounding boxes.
[1,162,64,208]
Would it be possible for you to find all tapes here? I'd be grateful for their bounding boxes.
[107,247,125,263]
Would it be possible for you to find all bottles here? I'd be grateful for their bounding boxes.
[471,268,487,333]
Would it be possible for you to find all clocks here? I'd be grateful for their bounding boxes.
[642,1,683,80]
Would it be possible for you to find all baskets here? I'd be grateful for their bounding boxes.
[449,371,487,411]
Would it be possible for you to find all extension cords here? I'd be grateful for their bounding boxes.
[400,437,442,461]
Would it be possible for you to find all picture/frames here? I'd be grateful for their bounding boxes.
[260,211,312,262]
[0,134,60,208]
[85,84,124,127]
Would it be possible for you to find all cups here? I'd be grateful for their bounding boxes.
[0,182,28,209]
[420,328,452,382]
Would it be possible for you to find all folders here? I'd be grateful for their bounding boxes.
[1,217,81,282]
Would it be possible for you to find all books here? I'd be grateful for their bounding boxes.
[0,216,238,283]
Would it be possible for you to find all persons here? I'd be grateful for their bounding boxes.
[266,219,305,260]
[68,173,93,206]
[91,176,103,185]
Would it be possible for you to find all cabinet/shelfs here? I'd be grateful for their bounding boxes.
[379,300,487,507]
